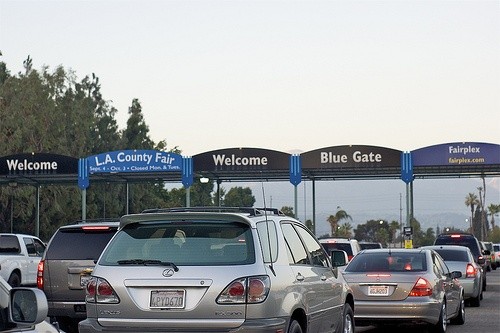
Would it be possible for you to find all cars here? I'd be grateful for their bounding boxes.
[341,247,466,333]
[479,240,500,271]
[418,246,486,308]
[0,274,66,333]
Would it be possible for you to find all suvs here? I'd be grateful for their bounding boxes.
[317,237,361,262]
[433,231,491,292]
[36,220,187,333]
[0,233,48,288]
[79,206,354,333]
[359,241,383,252]
